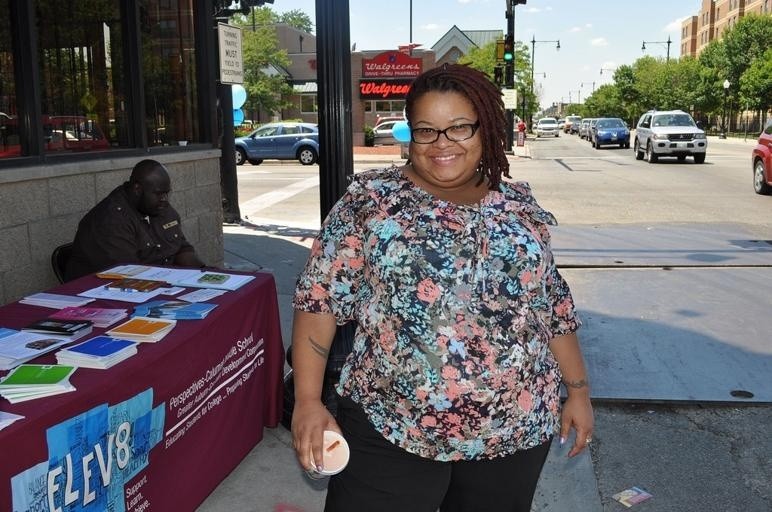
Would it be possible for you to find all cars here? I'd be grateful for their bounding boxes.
[235,121,319,168]
[367,121,402,146]
[514,115,522,128]
[591,118,631,150]
[0,112,116,159]
[751,116,772,195]
[531,112,598,145]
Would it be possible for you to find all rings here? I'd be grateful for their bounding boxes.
[586,437,592,442]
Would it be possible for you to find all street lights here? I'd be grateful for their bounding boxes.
[598,67,614,75]
[534,70,547,79]
[718,79,731,140]
[568,90,582,105]
[640,35,673,62]
[529,32,561,136]
[580,82,596,94]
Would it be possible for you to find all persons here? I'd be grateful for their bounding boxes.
[291,62,595,512]
[67,159,203,283]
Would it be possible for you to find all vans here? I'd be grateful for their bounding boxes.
[375,116,405,126]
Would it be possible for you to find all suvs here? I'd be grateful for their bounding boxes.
[632,107,708,164]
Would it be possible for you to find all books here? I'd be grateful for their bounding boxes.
[0,265,256,432]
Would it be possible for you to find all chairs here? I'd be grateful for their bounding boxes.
[51,241,77,284]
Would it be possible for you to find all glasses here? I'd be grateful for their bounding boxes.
[407,118,479,145]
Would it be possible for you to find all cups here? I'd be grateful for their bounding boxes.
[300,432,351,483]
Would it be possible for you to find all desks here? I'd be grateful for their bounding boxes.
[0,260,285,512]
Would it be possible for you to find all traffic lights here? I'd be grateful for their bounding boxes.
[504,48,514,65]
